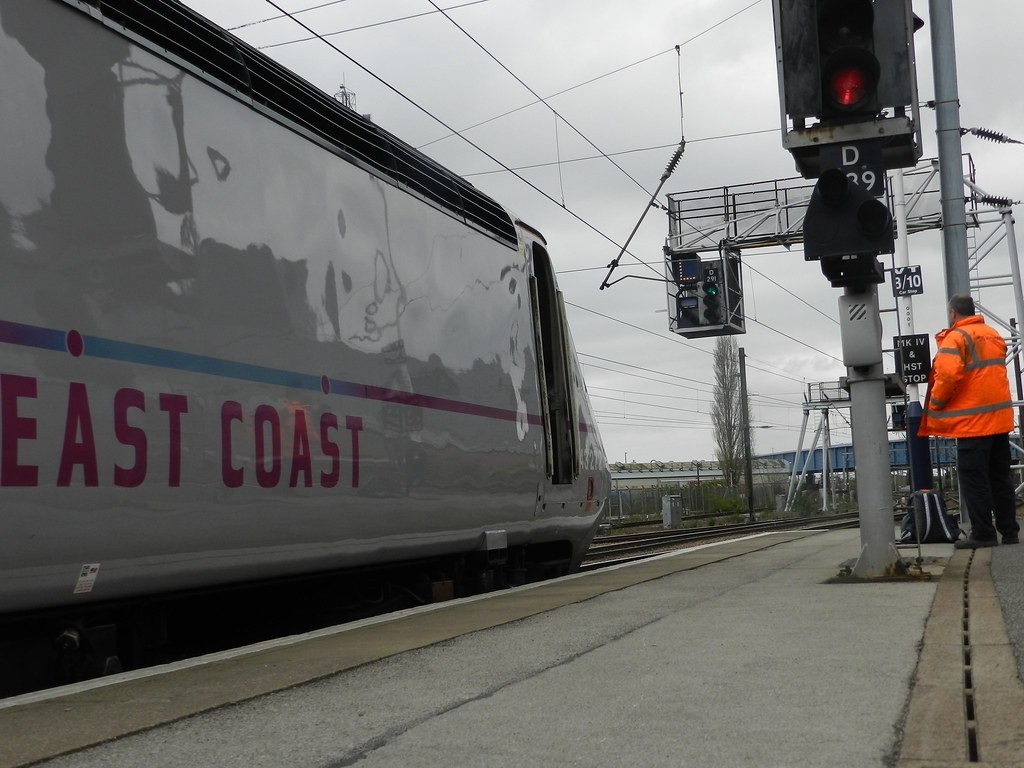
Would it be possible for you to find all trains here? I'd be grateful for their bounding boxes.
[1,0,610,699]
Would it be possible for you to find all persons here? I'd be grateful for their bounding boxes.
[918,294,1021,548]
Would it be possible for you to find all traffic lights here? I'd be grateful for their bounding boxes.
[772,0,915,118]
[670,252,702,283]
[802,167,895,261]
[680,297,700,318]
[702,268,720,321]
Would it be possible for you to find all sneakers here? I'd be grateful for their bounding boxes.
[953,535,997,549]
[1002,534,1020,543]
